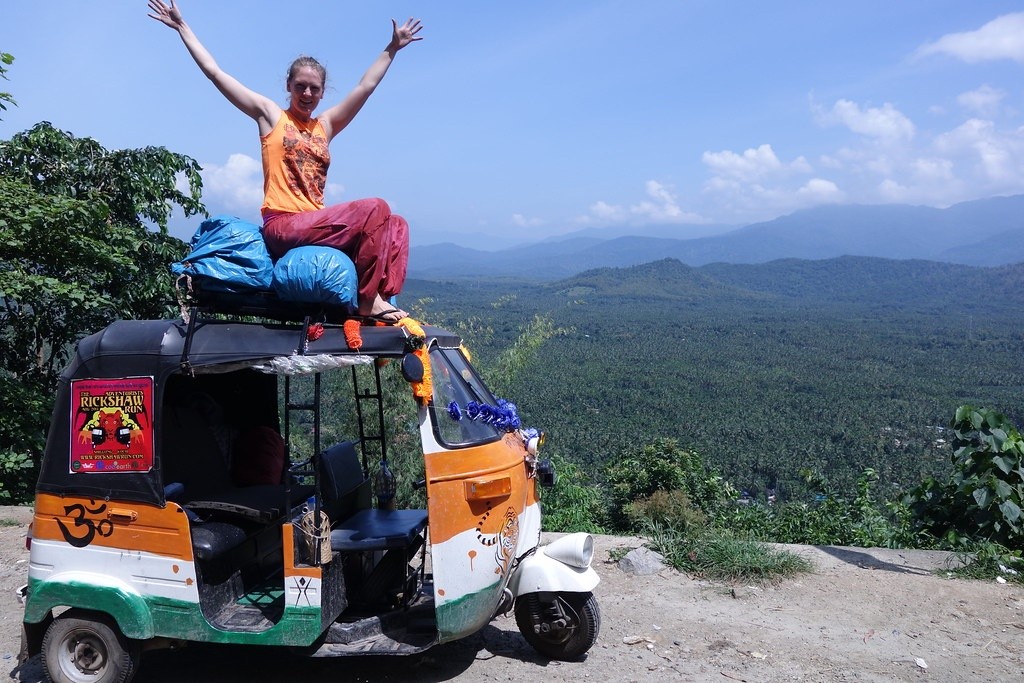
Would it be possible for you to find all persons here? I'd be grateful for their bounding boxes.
[146,0,424,324]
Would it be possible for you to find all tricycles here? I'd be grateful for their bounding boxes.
[11,317,605,683]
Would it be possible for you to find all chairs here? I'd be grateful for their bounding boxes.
[315,439,429,608]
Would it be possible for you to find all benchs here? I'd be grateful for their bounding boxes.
[155,374,315,614]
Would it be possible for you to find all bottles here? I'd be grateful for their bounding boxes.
[376,461,394,509]
[289,475,304,484]
[305,497,324,553]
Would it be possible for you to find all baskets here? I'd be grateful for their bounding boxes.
[373,467,397,510]
[301,510,333,565]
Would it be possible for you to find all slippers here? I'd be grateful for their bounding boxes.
[359,306,409,323]
